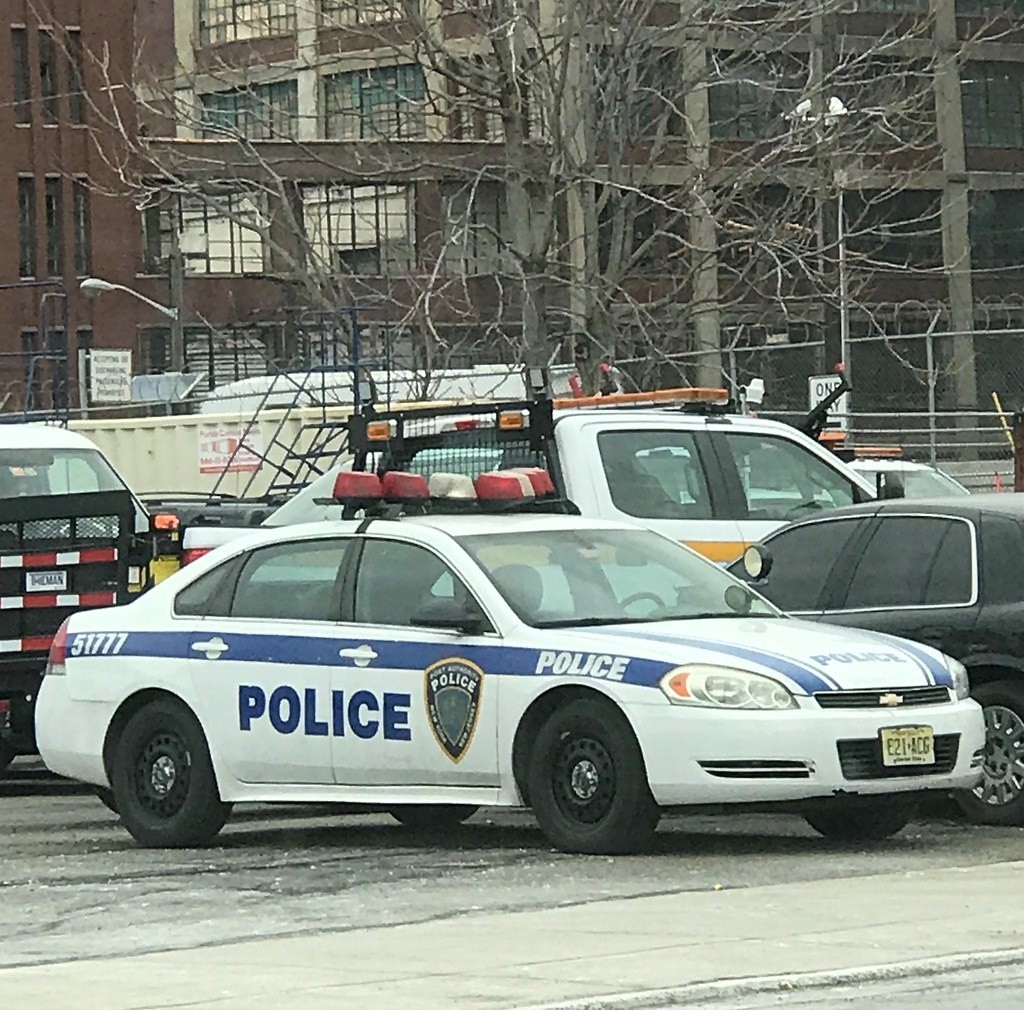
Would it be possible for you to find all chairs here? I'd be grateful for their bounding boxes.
[369,551,435,624]
[488,564,545,620]
[625,474,675,512]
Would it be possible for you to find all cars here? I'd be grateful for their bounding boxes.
[0,355,973,772]
[33,464,989,858]
[674,489,1023,826]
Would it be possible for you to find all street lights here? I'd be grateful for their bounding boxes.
[81,248,188,415]
[780,97,847,374]
